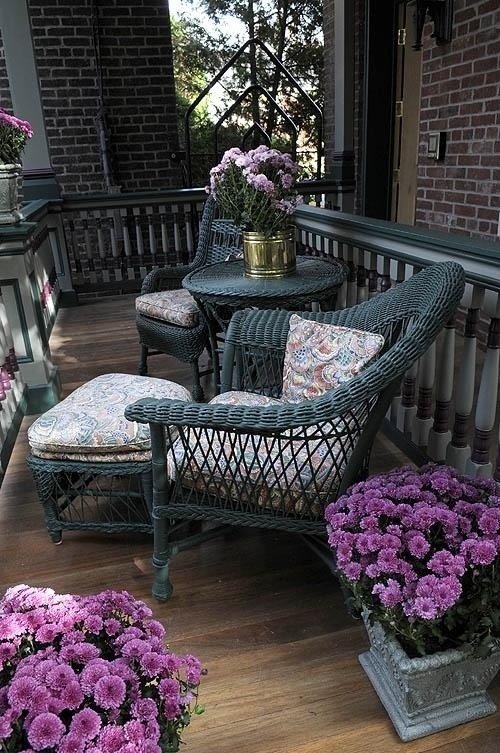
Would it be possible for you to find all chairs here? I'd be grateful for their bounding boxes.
[125,260,466,603]
[134,185,248,405]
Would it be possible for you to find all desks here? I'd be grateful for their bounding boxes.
[181,253,350,401]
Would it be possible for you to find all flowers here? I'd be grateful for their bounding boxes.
[1,106,34,160]
[204,143,310,233]
[1,580,208,753]
[322,458,499,663]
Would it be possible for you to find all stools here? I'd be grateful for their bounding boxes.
[24,367,199,541]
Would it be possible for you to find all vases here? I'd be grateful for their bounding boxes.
[1,164,23,226]
[353,606,499,747]
[240,226,299,277]
